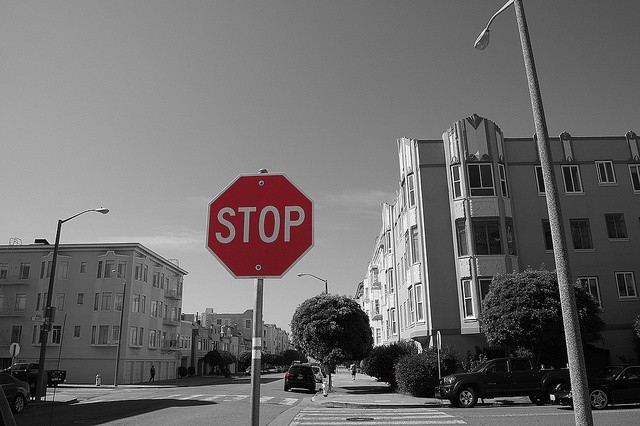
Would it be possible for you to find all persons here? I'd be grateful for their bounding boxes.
[350,364,357,380]
[149,365,155,382]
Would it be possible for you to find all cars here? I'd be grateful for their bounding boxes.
[245,366,251,376]
[0,373,30,414]
[550,365,640,409]
[246,366,251,376]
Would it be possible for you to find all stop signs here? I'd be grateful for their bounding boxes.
[206,173,315,279]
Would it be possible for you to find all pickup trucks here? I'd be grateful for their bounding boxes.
[266,366,278,374]
[440,357,569,408]
[3,363,66,387]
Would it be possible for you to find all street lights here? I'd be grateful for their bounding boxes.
[298,273,328,294]
[473,0,594,426]
[112,270,126,386]
[35,209,109,403]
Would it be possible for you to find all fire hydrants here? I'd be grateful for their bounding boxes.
[96,375,101,385]
[322,382,327,397]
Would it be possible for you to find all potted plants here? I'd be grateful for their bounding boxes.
[179,366,188,379]
[188,366,195,377]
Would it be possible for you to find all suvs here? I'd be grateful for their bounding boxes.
[320,367,326,378]
[285,365,316,391]
[311,366,324,383]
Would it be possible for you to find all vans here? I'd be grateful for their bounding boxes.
[277,365,284,373]
